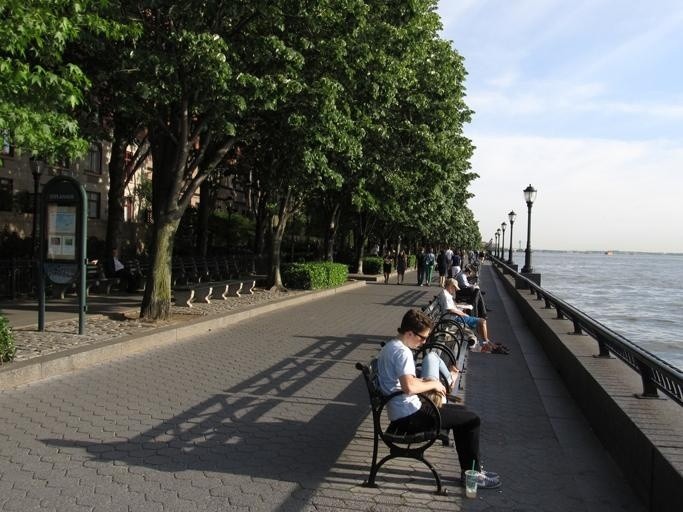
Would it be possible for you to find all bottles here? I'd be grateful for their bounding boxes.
[482,340,487,351]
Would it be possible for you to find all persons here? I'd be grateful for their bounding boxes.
[368,244,513,489]
[91,247,140,295]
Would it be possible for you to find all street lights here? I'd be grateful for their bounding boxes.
[494,232,498,256]
[224,195,234,225]
[520,184,537,274]
[506,210,517,264]
[29,152,48,258]
[500,221,507,259]
[496,228,501,258]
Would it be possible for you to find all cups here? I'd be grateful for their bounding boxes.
[464,470,480,498]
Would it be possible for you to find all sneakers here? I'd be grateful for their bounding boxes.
[481,342,510,354]
[461,469,502,488]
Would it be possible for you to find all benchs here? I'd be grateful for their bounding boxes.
[354,254,485,497]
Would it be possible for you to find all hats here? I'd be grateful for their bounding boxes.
[448,278,461,291]
